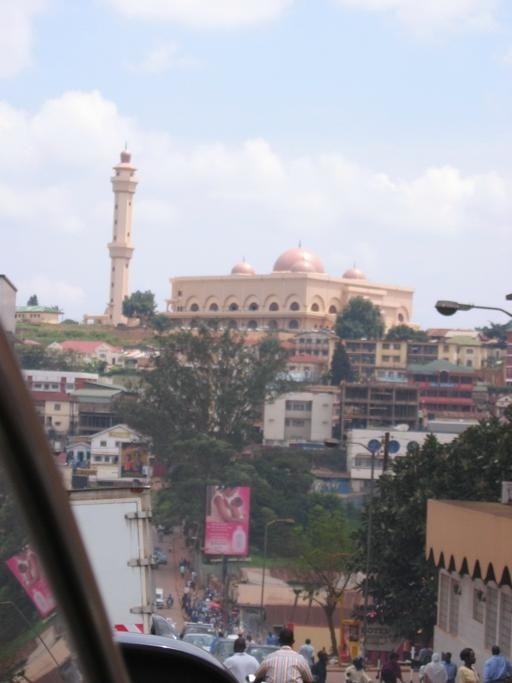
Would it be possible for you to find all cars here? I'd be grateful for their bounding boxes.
[150,551,167,569]
[179,622,281,663]
[156,588,165,609]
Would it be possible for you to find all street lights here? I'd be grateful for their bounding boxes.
[259,518,296,607]
[435,300,511,317]
[323,437,375,668]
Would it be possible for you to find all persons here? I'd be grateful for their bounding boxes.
[209,482,243,523]
[224,629,512,683]
[168,560,243,655]
[13,550,44,585]
